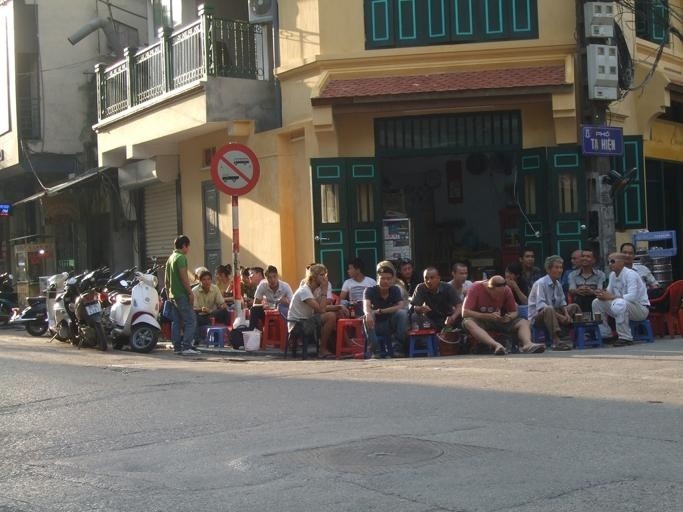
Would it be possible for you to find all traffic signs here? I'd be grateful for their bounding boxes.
[579,123,625,158]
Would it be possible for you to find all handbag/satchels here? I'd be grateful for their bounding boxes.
[229,325,255,349]
[162,299,173,321]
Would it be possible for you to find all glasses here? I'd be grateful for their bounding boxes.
[607,260,616,264]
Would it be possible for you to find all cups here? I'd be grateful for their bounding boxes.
[209,318,215,327]
[595,312,601,321]
[575,313,583,321]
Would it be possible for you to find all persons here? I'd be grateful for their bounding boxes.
[160,234,202,358]
[194,243,665,357]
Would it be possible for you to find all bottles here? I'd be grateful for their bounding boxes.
[348,303,355,319]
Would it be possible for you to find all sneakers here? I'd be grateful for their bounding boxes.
[371,351,385,358]
[391,349,406,358]
[174,349,201,356]
[603,336,633,345]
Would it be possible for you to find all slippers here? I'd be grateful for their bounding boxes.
[318,353,337,359]
[527,340,573,354]
[494,346,507,355]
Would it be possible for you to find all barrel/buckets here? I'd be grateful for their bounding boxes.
[436,331,462,356]
[242,331,260,351]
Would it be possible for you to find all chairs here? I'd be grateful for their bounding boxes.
[647,279,683,338]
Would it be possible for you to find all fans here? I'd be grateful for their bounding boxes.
[607,167,637,199]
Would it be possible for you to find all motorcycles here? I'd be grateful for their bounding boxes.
[0,261,163,357]
